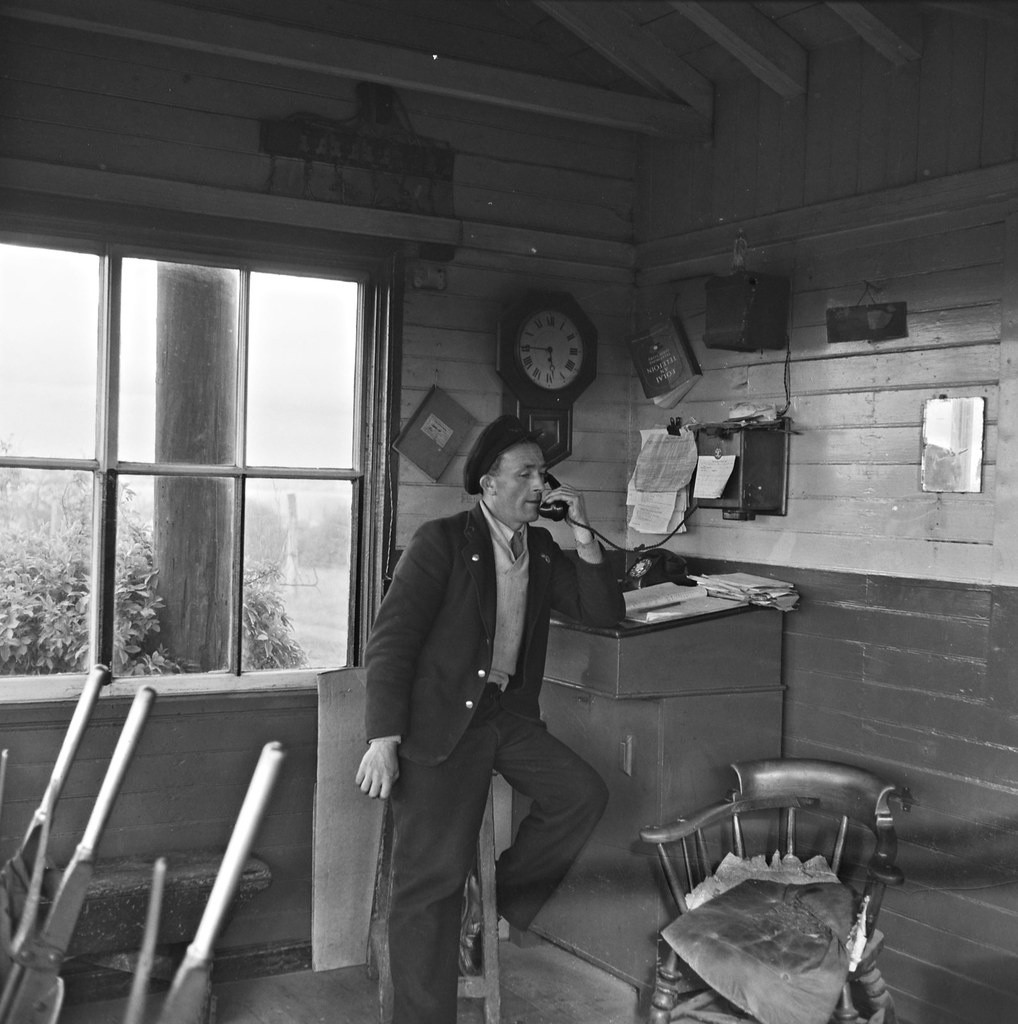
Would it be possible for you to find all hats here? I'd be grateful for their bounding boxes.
[464,414,544,495]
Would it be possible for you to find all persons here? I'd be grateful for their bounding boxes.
[354,413,627,1024]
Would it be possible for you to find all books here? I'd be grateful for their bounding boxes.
[623,582,749,624]
[625,316,704,410]
[392,384,477,484]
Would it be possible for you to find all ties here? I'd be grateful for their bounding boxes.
[512,531,524,560]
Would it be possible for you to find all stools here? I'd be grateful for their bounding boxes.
[365,771,503,1024]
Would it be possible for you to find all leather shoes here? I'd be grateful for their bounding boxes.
[457,863,501,978]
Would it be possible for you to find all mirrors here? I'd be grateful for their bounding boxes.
[921,396,986,493]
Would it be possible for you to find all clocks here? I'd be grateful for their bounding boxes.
[494,289,599,471]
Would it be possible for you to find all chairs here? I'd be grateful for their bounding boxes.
[639,760,916,1024]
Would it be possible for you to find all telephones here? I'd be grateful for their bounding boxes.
[538,471,569,522]
